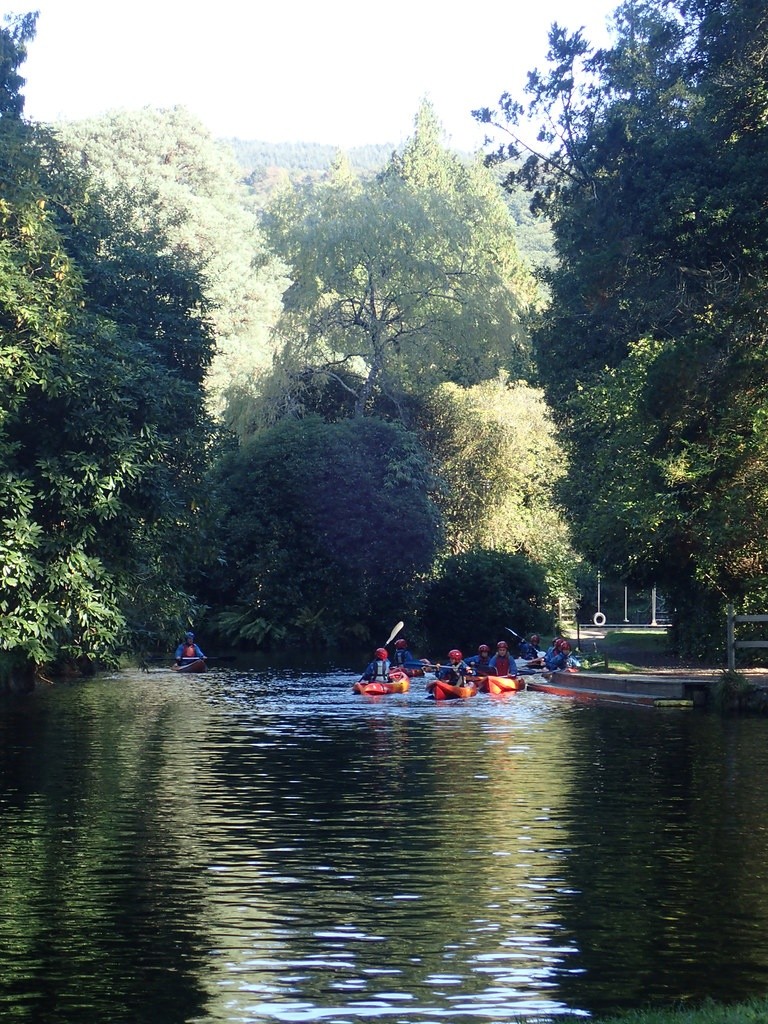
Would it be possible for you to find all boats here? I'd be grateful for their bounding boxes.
[389,658,433,678]
[466,651,578,694]
[350,671,410,695]
[424,679,478,700]
[171,659,208,673]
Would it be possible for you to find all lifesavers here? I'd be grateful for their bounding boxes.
[594,612,606,626]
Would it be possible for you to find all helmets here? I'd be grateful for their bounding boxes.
[395,639,407,649]
[479,645,489,654]
[556,639,563,648]
[448,650,462,663]
[561,641,570,652]
[531,635,540,644]
[375,648,388,660]
[185,632,195,641]
[552,637,560,646]
[497,641,508,648]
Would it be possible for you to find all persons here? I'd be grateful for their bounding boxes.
[362,648,393,683]
[175,632,207,666]
[465,633,581,679]
[435,650,476,687]
[394,639,431,670]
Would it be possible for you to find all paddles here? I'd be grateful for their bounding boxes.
[463,661,488,668]
[403,660,465,670]
[497,669,536,678]
[346,621,404,692]
[505,626,546,658]
